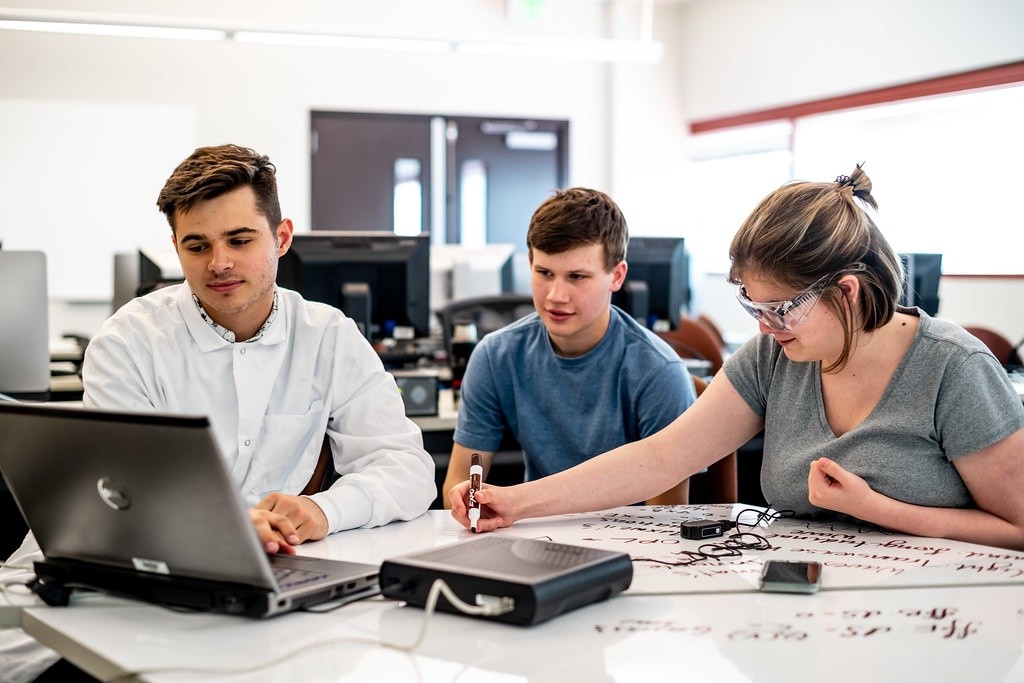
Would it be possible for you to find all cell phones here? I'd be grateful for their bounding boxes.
[759,560,823,594]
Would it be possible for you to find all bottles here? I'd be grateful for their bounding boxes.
[452,319,478,412]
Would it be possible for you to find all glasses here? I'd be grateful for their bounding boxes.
[737,260,868,329]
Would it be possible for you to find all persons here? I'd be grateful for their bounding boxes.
[0,142,437,683]
[449,161,1024,552]
[442,187,696,512]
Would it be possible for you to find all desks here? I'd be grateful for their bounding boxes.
[0,511,1023,683]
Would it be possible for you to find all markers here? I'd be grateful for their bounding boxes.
[468,452,480,531]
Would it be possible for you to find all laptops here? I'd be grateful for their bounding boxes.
[0,399,387,619]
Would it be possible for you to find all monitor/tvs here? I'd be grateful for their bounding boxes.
[0,250,50,393]
[611,237,685,334]
[113,250,161,315]
[896,253,943,317]
[276,230,430,356]
